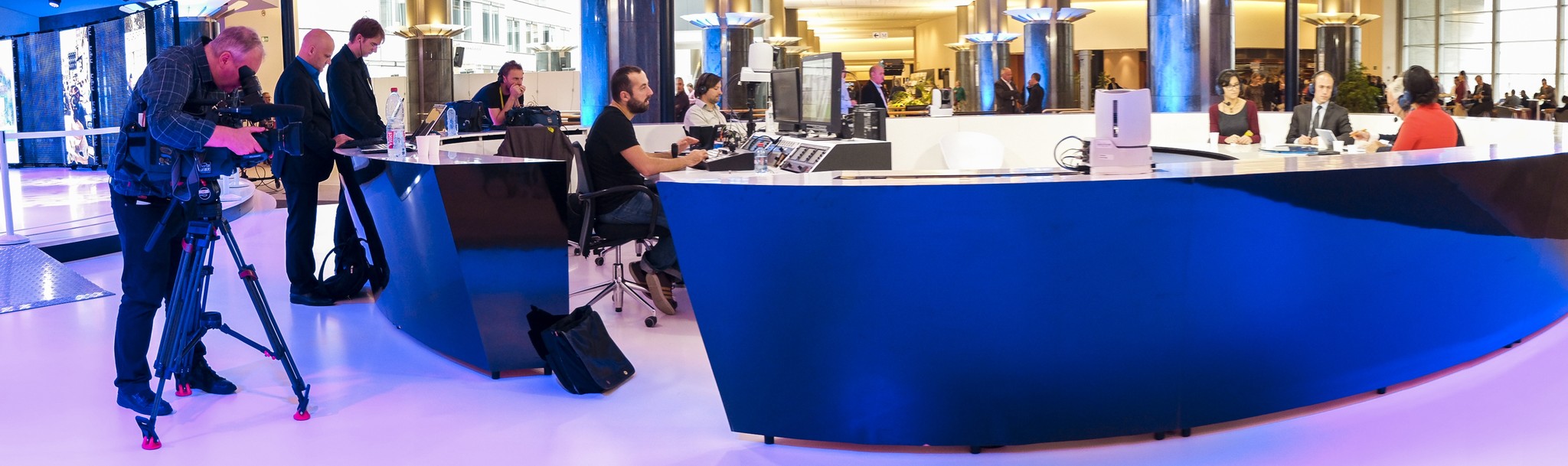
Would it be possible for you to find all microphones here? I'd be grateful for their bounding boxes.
[1219,99,1231,107]
[214,102,305,118]
[1394,108,1403,122]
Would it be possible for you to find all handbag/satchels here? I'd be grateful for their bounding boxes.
[505,104,563,126]
[540,305,635,394]
[443,99,485,132]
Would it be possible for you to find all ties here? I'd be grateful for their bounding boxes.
[1310,106,1322,138]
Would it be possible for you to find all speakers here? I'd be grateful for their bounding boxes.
[453,47,466,67]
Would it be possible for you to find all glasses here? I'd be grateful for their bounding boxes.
[1222,83,1240,91]
[1386,99,1398,107]
[369,40,381,49]
[264,97,272,99]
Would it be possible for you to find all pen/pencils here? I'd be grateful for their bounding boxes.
[1349,128,1367,137]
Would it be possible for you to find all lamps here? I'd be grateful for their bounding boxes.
[48,0,62,8]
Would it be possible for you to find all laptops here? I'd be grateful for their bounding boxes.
[339,98,404,149]
[356,105,448,153]
[800,52,842,134]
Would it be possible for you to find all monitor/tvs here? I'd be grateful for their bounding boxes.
[770,67,800,125]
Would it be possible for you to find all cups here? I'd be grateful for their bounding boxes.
[1332,140,1345,152]
[1348,145,1358,154]
[1317,136,1327,148]
[428,135,441,157]
[415,136,429,155]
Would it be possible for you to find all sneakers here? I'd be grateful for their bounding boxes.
[628,261,654,300]
[174,361,237,395]
[116,387,174,416]
[645,271,678,315]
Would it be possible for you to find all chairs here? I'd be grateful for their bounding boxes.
[1377,90,1568,122]
[567,141,664,331]
[495,120,595,260]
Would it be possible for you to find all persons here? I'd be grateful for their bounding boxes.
[108,25,275,420]
[469,60,528,127]
[50,53,137,167]
[1236,67,1568,122]
[327,16,388,274]
[682,73,728,138]
[1348,76,1465,153]
[583,64,709,316]
[1391,65,1459,151]
[1284,69,1354,148]
[685,83,696,108]
[258,93,275,131]
[1107,78,1123,90]
[672,77,691,124]
[1208,68,1261,146]
[271,28,354,309]
[839,58,1044,119]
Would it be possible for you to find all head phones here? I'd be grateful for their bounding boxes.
[1397,94,1410,112]
[1404,65,1440,103]
[1308,70,1338,97]
[1216,68,1243,95]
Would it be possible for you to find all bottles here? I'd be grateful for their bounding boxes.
[447,106,457,135]
[754,143,768,172]
[386,88,407,157]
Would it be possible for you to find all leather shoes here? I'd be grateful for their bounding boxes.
[290,283,337,306]
